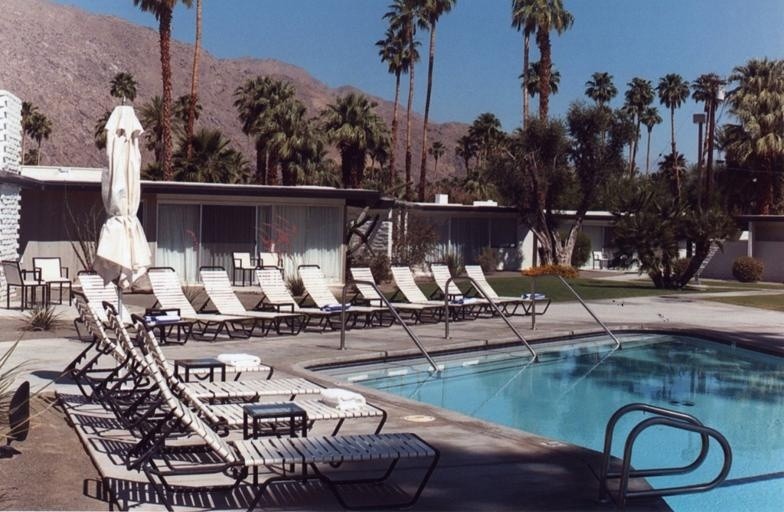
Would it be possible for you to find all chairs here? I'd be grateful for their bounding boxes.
[431,264,501,320]
[146,267,251,343]
[298,266,388,329]
[59,292,330,421]
[141,353,439,509]
[32,257,73,306]
[466,264,548,315]
[349,266,438,325]
[78,272,134,343]
[200,266,306,335]
[126,326,390,470]
[390,266,465,322]
[251,269,332,332]
[3,260,45,311]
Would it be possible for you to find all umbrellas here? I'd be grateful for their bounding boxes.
[89,104,152,338]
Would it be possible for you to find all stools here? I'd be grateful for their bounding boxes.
[441,292,466,320]
[355,297,382,328]
[262,300,295,335]
[146,306,181,344]
